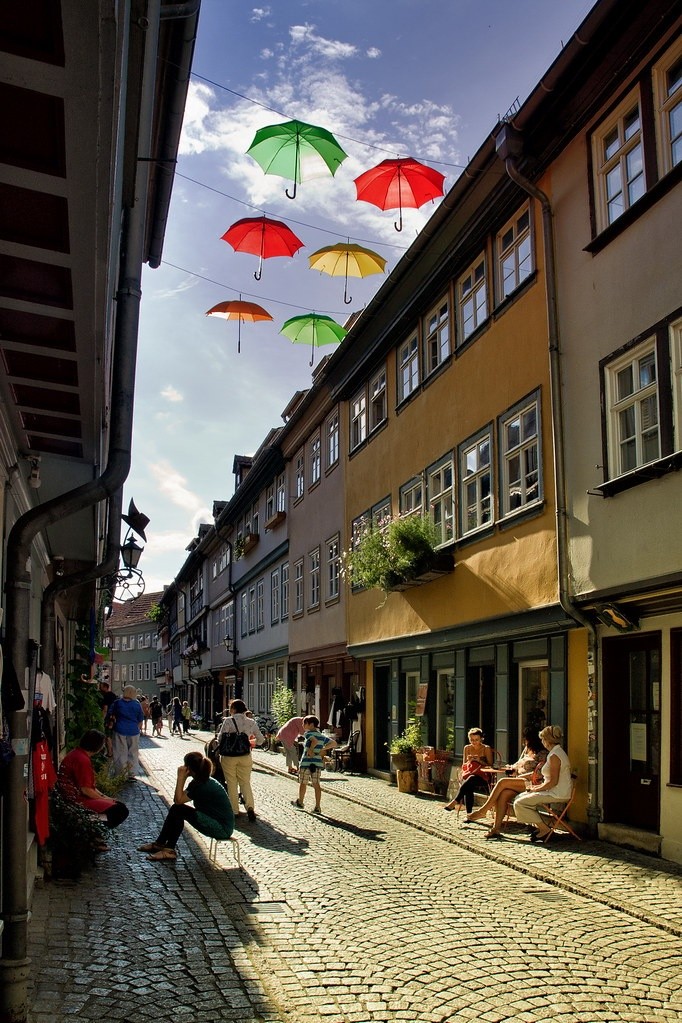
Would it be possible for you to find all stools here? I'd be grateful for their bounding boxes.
[209,835,241,870]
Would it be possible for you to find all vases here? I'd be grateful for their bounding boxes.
[384,550,453,591]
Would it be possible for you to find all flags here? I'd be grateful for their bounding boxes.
[94,648,110,664]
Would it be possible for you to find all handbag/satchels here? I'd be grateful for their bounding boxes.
[310,757,316,773]
[108,699,119,729]
[462,756,492,784]
[220,715,251,757]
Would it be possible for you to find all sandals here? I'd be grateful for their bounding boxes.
[146,848,177,860]
[288,767,300,773]
[466,810,486,820]
[484,828,500,838]
[136,842,166,852]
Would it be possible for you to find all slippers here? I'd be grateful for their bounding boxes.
[105,754,113,758]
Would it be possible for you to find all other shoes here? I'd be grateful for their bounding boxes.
[530,827,552,843]
[128,777,137,782]
[290,798,304,809]
[234,813,241,819]
[463,818,473,823]
[310,807,321,815]
[444,804,456,811]
[100,844,110,851]
[248,807,256,823]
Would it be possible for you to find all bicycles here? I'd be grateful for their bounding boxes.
[259,723,272,753]
[215,709,230,736]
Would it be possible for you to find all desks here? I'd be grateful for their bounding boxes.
[479,765,516,794]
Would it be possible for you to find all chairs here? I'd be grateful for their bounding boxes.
[502,761,545,832]
[456,750,503,819]
[333,730,360,774]
[538,779,582,843]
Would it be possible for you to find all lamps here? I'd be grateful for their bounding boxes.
[96,532,145,590]
[223,634,239,655]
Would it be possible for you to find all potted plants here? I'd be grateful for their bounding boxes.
[233,533,260,562]
[383,718,423,770]
[426,750,452,795]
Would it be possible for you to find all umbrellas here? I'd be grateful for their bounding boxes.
[205,294,274,354]
[219,212,306,281]
[307,236,387,304]
[353,154,447,232]
[279,312,348,366]
[244,118,348,199]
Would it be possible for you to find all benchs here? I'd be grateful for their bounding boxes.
[80,810,110,823]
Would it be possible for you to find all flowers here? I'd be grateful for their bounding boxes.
[330,504,441,609]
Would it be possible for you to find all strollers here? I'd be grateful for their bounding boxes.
[204,737,245,806]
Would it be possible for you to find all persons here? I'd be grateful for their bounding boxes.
[445,728,493,823]
[108,685,144,781]
[137,752,235,860]
[514,725,573,842]
[59,729,130,852]
[137,695,191,738]
[467,726,547,837]
[218,699,264,823]
[99,681,118,757]
[531,700,546,728]
[276,715,337,814]
